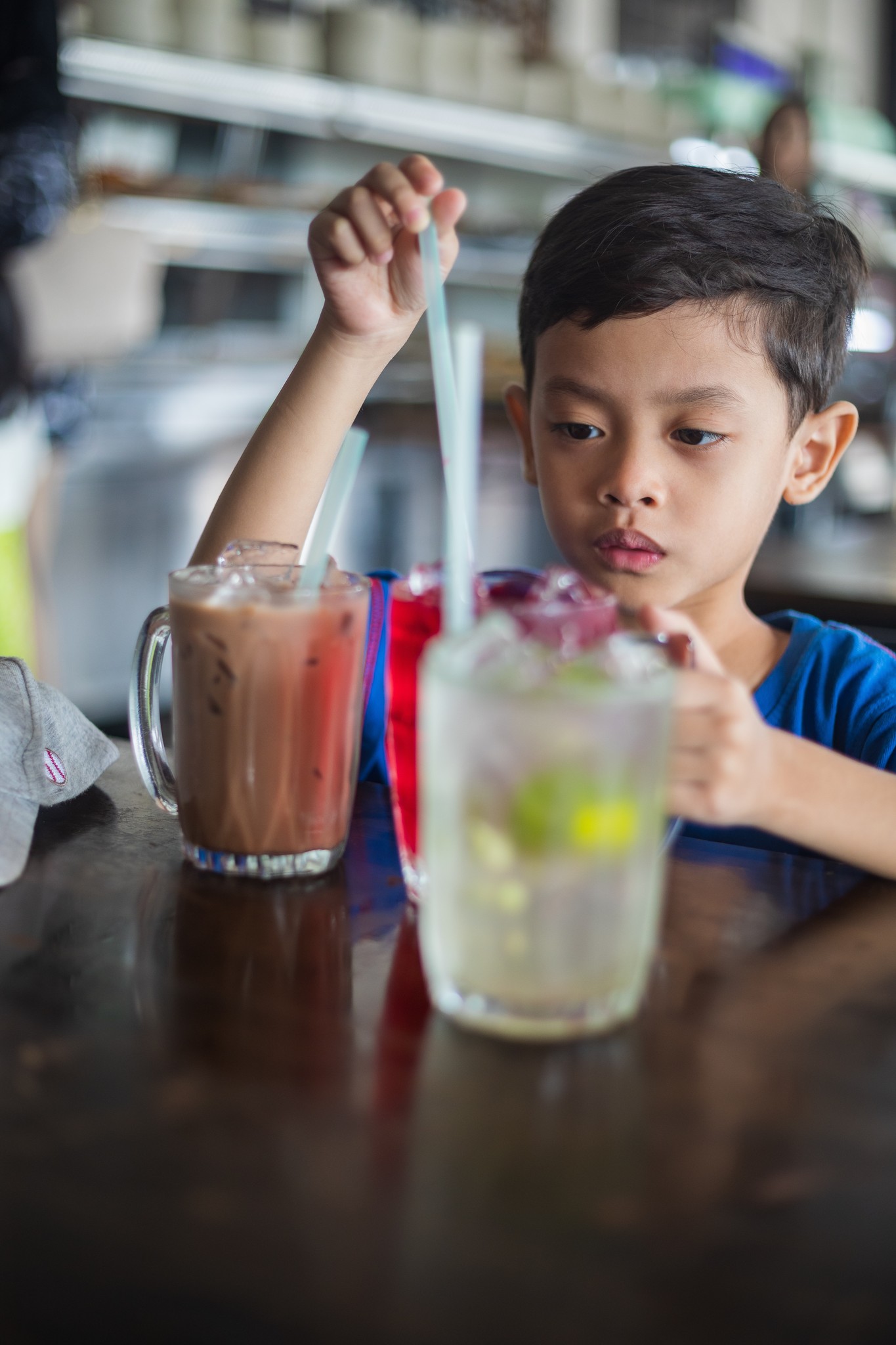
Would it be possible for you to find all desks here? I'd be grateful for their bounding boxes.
[0,725,896,1345]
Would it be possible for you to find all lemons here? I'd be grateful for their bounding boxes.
[511,781,639,855]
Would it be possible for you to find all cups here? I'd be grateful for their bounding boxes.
[127,566,371,879]
[417,631,696,1044]
[382,566,622,902]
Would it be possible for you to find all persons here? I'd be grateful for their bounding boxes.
[0,0,82,685]
[752,89,824,196]
[184,154,896,885]
